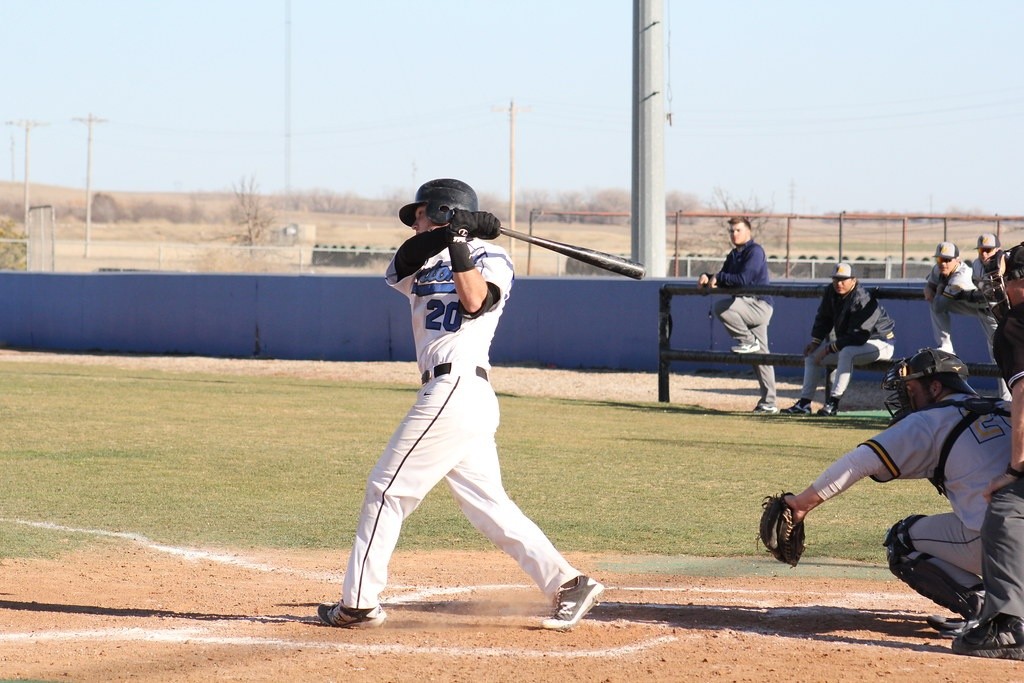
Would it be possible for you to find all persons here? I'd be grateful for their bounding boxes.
[924,233,1012,402]
[780,263,896,416]
[950,241,1024,661]
[317,179,606,633]
[699,216,778,414]
[759,347,1011,640]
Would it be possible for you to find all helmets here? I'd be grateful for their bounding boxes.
[906,347,979,395]
[399,179,478,226]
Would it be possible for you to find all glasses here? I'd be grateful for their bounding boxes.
[936,257,952,263]
[978,248,994,253]
[832,276,851,280]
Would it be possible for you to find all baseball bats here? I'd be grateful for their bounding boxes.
[448,209,646,282]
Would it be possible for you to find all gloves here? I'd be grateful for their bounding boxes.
[472,212,502,240]
[445,209,477,244]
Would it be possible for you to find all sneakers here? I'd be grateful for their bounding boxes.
[818,403,839,415]
[541,576,605,629]
[731,338,761,353]
[780,401,811,414]
[316,599,386,628]
[950,618,1024,660]
[752,406,779,414]
[927,616,974,638]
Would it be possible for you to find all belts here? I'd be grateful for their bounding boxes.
[421,363,488,385]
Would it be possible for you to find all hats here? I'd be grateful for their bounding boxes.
[974,234,1001,249]
[932,241,959,258]
[831,262,857,278]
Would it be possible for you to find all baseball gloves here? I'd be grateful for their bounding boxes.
[755,489,806,569]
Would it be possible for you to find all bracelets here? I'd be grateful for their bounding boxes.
[1005,462,1023,477]
